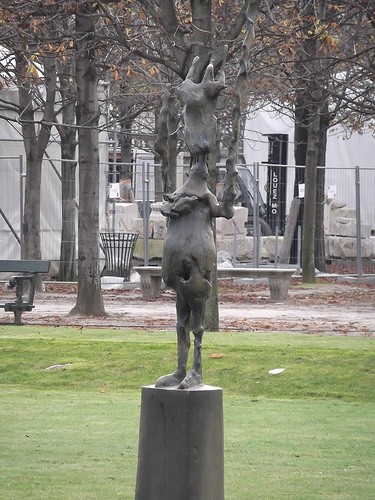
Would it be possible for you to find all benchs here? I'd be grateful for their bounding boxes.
[132,265,297,302]
[0,259,51,326]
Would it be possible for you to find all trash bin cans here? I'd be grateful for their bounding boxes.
[99,232,140,278]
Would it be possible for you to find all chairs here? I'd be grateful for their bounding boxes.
[98,232,139,284]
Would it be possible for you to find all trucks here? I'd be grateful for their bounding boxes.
[106,145,273,236]
